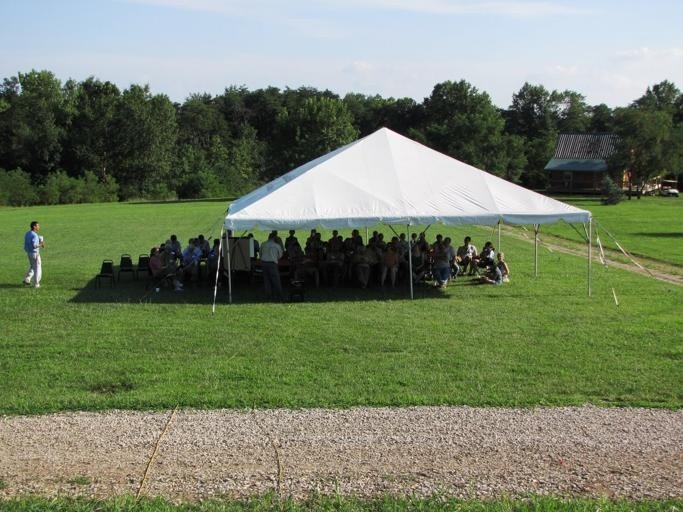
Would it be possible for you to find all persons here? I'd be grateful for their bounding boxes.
[148,228,458,292]
[22,220,46,289]
[458,237,509,285]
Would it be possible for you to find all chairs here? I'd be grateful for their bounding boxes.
[94,258,116,289]
[136,253,151,282]
[151,247,494,288]
[117,254,135,284]
